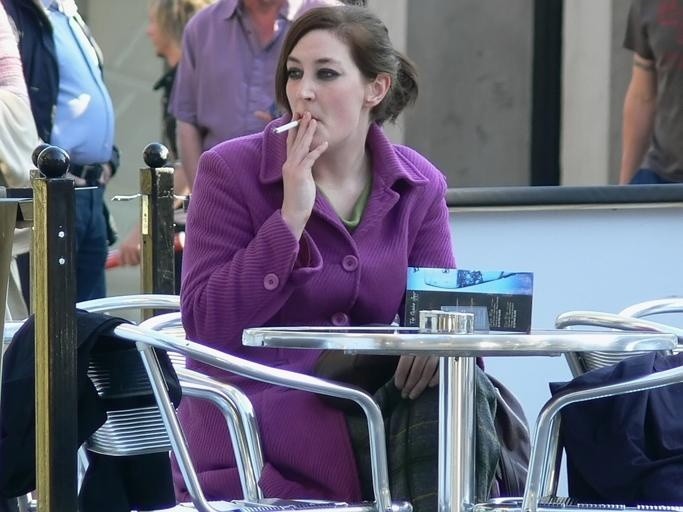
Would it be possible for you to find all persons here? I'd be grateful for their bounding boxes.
[168,4,503,511]
[115,0,209,313]
[614,2,682,185]
[166,0,348,194]
[0,6,41,183]
[0,0,120,350]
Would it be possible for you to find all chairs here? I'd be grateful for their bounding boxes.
[618,298,683,318]
[554,311,683,379]
[473,349,683,512]
[1,294,187,512]
[113,312,414,512]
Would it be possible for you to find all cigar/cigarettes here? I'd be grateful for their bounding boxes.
[272,117,303,136]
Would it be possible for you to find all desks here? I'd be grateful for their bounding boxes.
[241,326,679,512]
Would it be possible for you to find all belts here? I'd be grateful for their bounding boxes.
[65,161,116,181]
